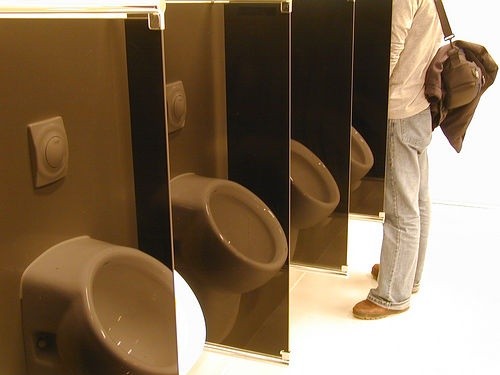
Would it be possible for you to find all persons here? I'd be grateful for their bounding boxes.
[353,0,443,319]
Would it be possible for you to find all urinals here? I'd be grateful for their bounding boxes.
[169,171,288,345]
[351,123,375,195]
[20,234,179,375]
[290,139,341,263]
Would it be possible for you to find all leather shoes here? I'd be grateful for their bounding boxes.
[370,264,419,293]
[353,299,410,321]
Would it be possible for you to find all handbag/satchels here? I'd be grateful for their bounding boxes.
[425,0,499,153]
